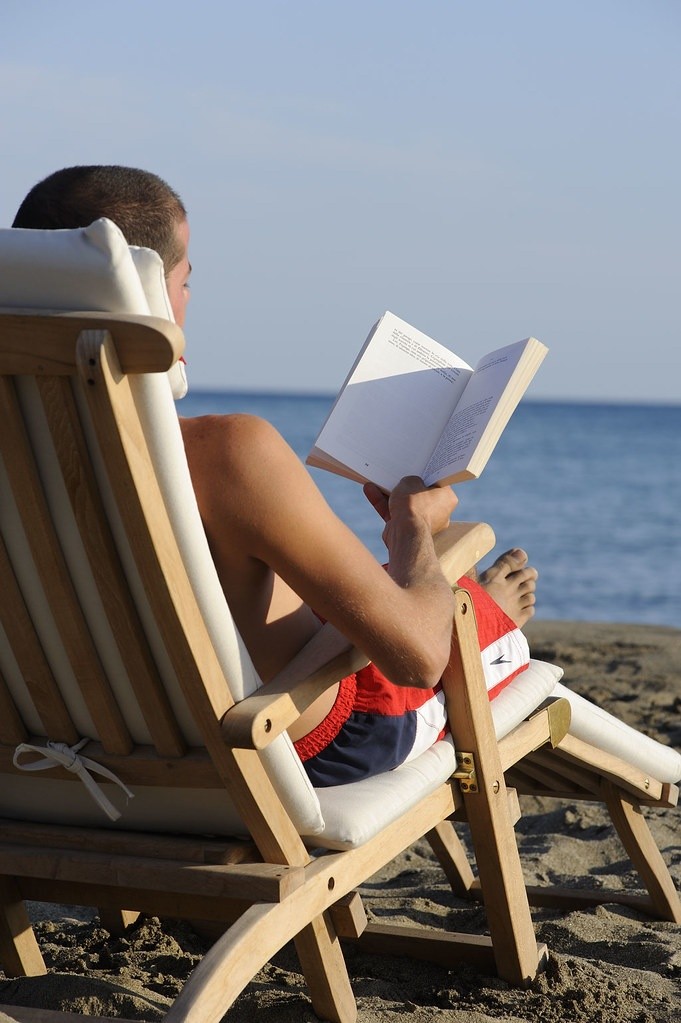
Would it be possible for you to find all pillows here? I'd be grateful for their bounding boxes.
[128,246,188,400]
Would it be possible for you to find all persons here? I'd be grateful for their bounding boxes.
[9,161,538,792]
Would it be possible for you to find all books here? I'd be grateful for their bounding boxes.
[303,310,550,491]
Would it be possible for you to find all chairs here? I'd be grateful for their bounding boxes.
[1,216,681,1023]
